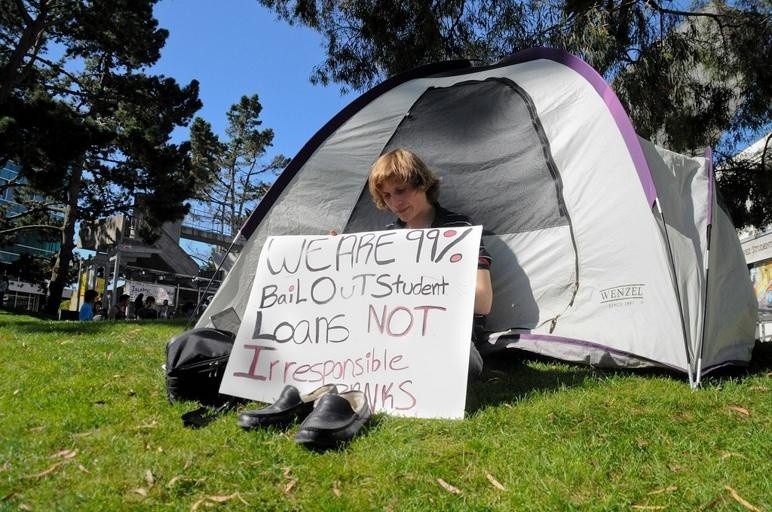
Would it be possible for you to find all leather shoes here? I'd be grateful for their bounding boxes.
[238,383,340,431]
[293,390,371,451]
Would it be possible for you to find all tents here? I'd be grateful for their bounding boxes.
[191,48,762,395]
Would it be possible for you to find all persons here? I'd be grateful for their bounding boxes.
[327,153,496,393]
[74,284,175,319]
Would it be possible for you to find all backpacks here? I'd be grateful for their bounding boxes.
[164,327,237,405]
